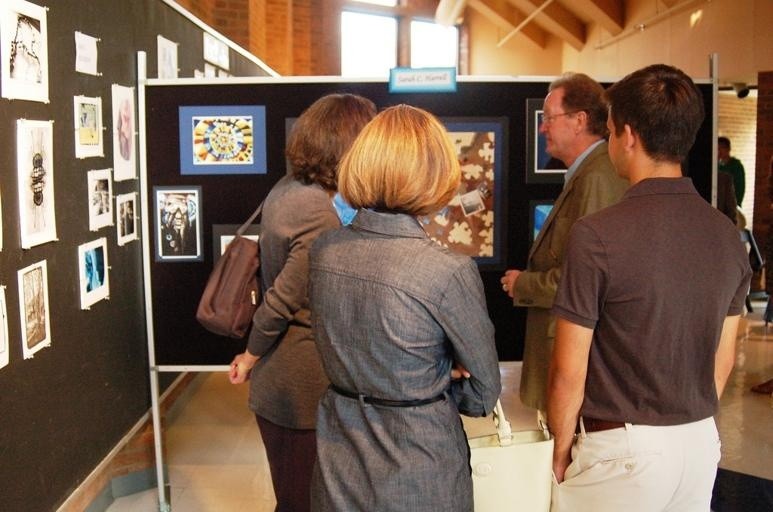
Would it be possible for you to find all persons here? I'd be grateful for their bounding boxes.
[718,170,737,226]
[308,103,502,512]
[549,63,753,512]
[227,93,378,512]
[499,72,629,441]
[718,137,746,208]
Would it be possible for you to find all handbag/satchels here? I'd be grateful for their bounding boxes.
[466,399,555,511]
[194,234,270,340]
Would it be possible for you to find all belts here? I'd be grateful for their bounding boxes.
[572,413,626,435]
[325,381,452,409]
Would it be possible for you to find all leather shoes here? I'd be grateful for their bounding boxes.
[750,378,773,394]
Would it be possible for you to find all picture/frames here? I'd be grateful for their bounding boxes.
[153,186,205,263]
[211,224,259,269]
[417,113,510,273]
[525,98,567,184]
[529,199,556,252]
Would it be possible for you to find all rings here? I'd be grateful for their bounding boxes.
[503,283,508,292]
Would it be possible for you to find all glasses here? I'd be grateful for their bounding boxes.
[538,108,586,124]
